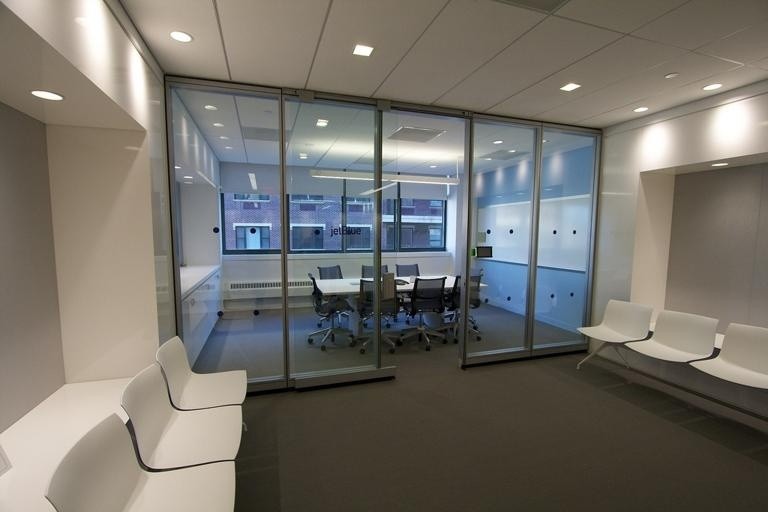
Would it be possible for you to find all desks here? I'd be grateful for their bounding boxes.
[312,275,489,338]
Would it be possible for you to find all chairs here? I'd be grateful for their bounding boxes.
[576,299,768,389]
[308,263,481,355]
[44,335,248,512]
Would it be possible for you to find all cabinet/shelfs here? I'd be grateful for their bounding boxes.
[182,264,224,369]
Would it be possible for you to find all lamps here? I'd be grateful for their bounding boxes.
[309,149,461,186]
